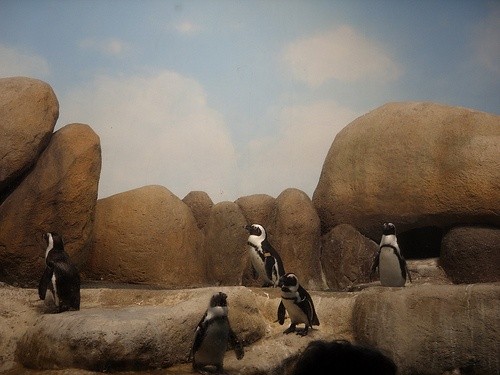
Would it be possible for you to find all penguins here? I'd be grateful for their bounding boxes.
[277,273,320,337]
[188,292,244,375]
[240,224,285,289]
[370,222,413,286]
[39,231,80,313]
